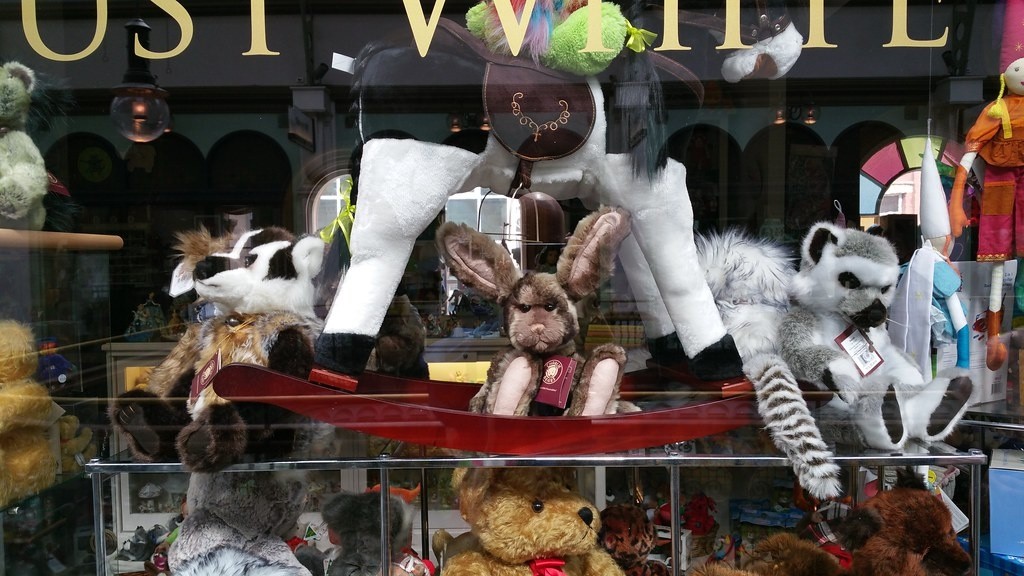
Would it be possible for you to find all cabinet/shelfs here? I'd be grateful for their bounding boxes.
[106,320,584,551]
[81,413,986,576]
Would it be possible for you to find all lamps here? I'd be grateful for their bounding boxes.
[108,16,171,145]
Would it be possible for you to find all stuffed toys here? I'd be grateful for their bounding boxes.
[96,458,987,576]
[463,0,655,77]
[2,319,98,513]
[949,42,1024,369]
[114,224,322,474]
[317,0,804,418]
[697,219,974,501]
[894,134,973,381]
[0,62,50,231]
[431,205,646,420]
